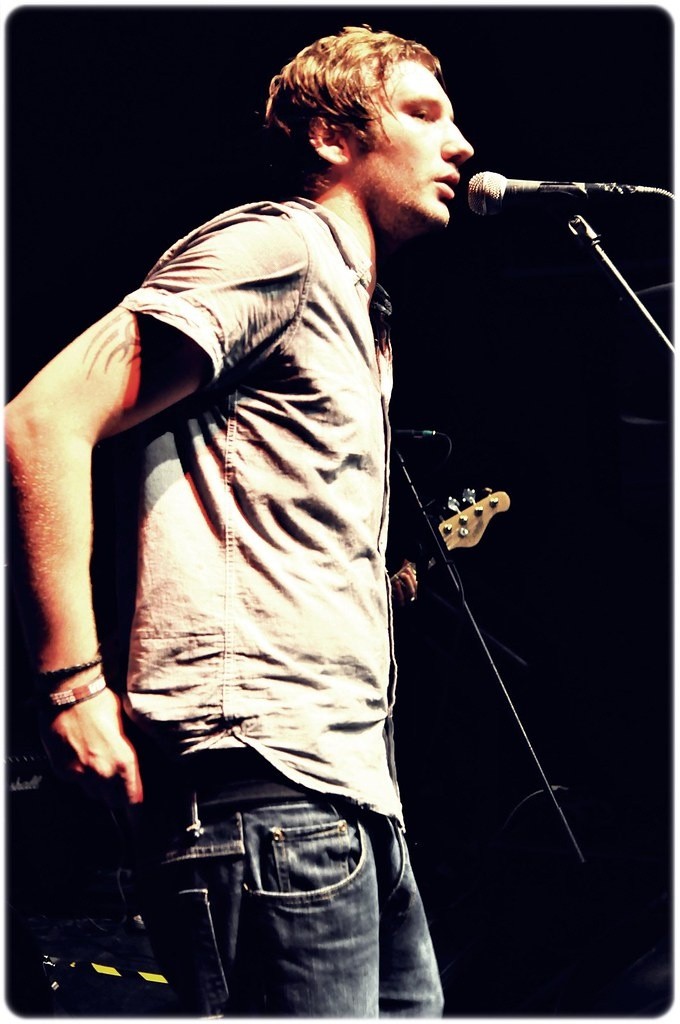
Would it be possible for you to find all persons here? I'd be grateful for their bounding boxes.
[5,23,474,1019]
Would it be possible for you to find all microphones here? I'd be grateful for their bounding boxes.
[468,171,636,214]
[391,430,437,439]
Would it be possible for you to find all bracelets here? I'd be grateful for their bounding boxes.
[36,656,103,693]
[49,673,107,707]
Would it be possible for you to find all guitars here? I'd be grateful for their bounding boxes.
[388,487,512,608]
